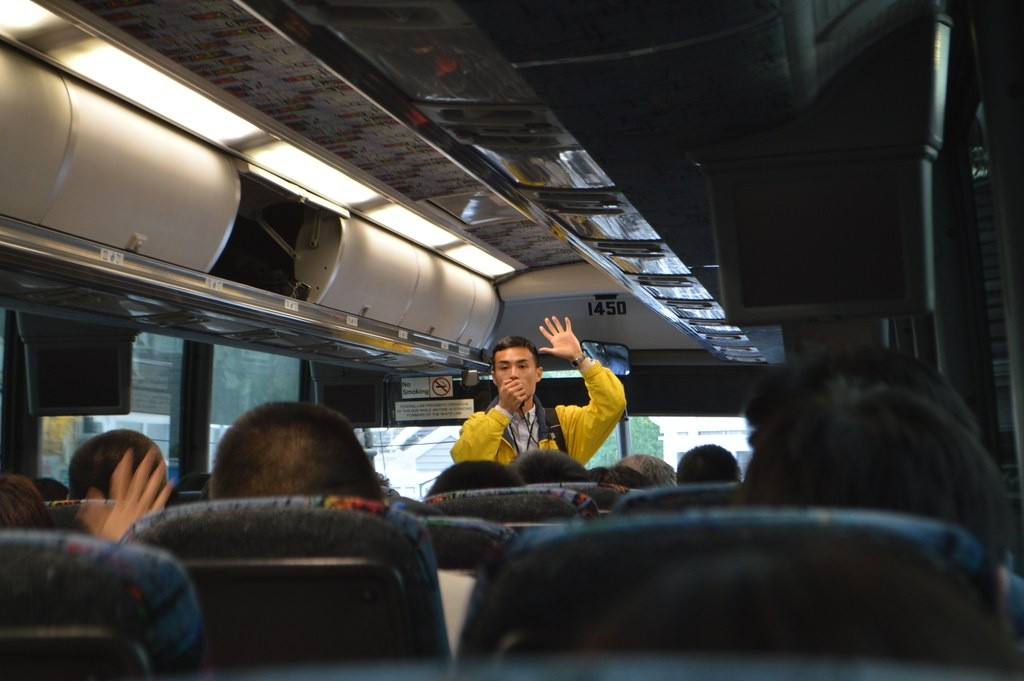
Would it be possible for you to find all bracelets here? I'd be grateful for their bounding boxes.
[571,351,588,367]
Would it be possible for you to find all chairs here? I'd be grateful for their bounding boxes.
[0,478,1023,681]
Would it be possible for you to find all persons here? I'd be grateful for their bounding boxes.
[428,344,1010,620]
[451,315,624,467]
[0,402,383,540]
